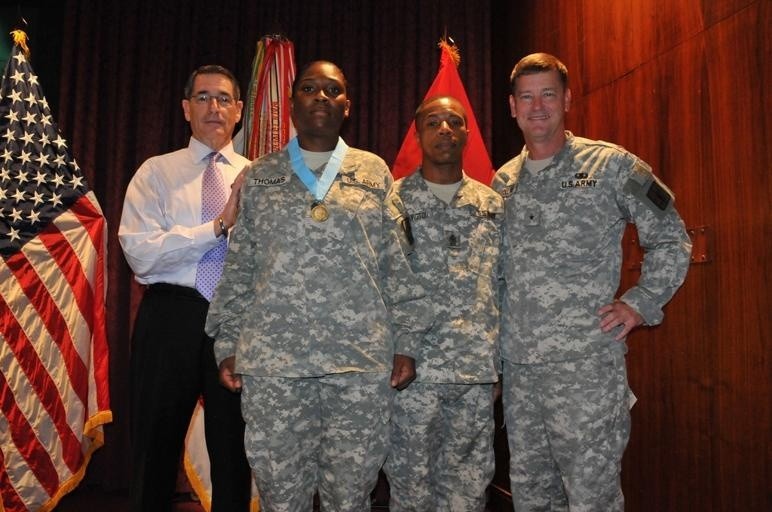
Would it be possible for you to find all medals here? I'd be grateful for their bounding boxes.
[311,204,329,222]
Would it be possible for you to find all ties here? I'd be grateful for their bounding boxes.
[195,152,227,303]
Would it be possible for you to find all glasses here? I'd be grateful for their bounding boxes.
[189,93,237,105]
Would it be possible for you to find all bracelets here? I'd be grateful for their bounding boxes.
[217,217,227,239]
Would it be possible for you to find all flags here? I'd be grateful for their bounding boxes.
[182,32,297,511]
[391,38,496,187]
[0,27,114,512]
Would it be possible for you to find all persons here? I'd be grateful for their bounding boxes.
[383,93,504,511]
[487,53,694,512]
[118,64,255,511]
[204,58,394,511]
